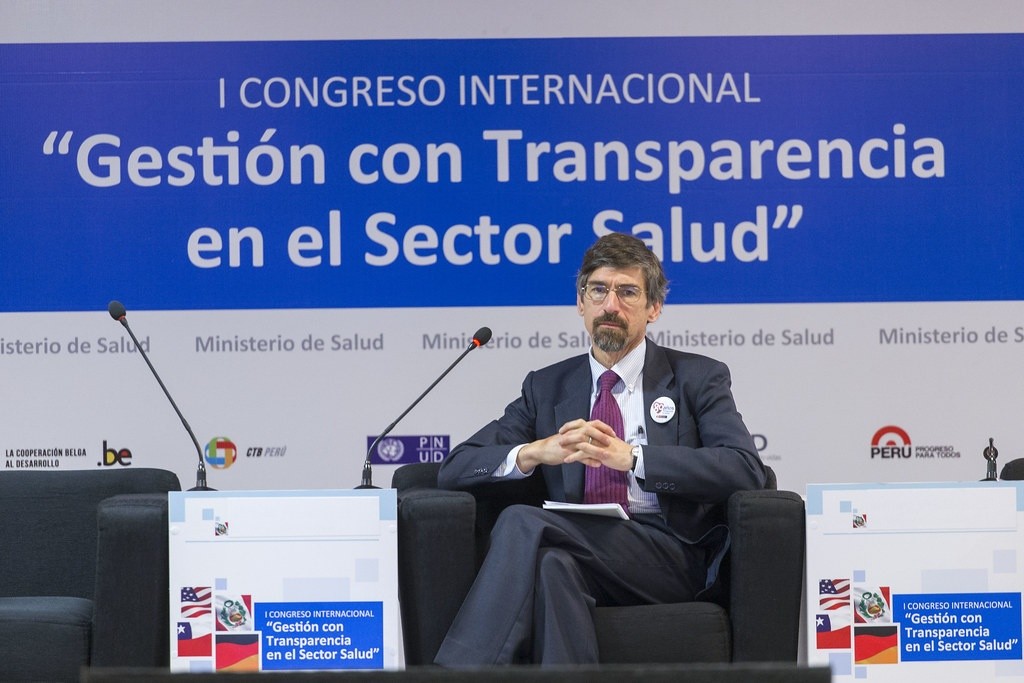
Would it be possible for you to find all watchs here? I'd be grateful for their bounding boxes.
[631,445,640,473]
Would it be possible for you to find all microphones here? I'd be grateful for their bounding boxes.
[354,327,492,490]
[107,300,217,492]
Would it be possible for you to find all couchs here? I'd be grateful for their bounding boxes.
[391,462,807,664]
[0,468,182,683]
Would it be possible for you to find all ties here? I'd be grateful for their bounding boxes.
[584,370,629,516]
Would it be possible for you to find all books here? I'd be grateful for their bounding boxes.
[542,500,630,520]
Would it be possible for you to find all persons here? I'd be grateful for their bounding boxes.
[433,232,770,668]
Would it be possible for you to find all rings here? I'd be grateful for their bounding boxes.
[588,435,593,444]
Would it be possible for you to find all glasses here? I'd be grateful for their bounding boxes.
[580,283,650,303]
